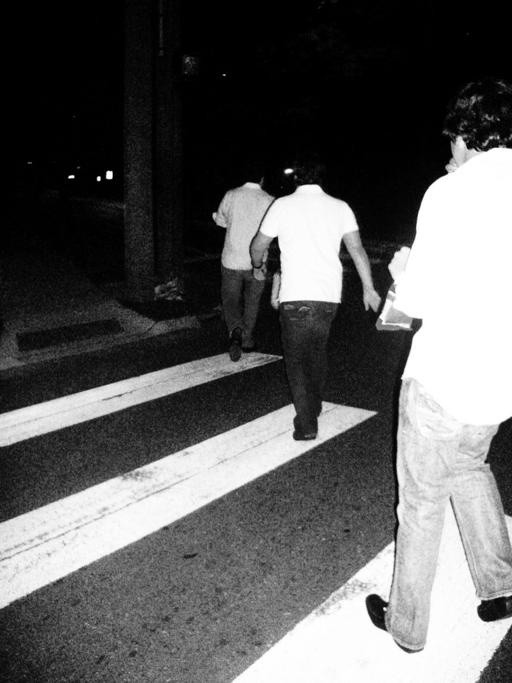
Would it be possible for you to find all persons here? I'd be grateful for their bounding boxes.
[363,74,512,655]
[249,146,382,440]
[211,161,274,360]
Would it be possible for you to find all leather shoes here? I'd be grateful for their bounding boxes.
[293,424,315,441]
[475,592,512,622]
[365,592,424,653]
[241,345,255,354]
[229,326,243,362]
